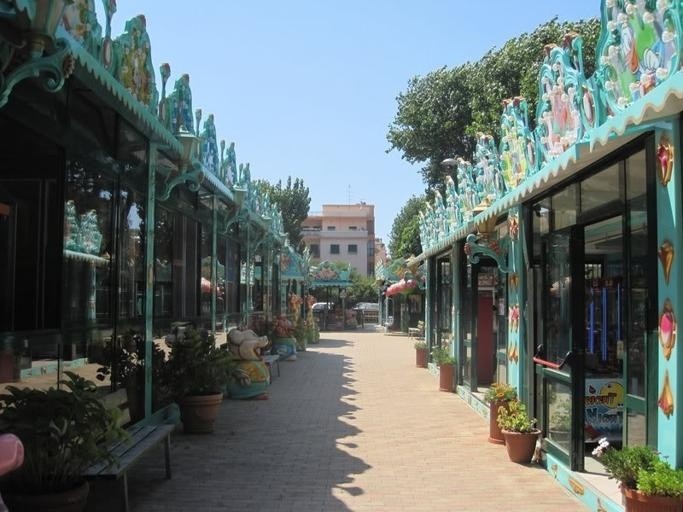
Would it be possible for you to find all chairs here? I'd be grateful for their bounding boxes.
[257,346,280,384]
[407,320,425,342]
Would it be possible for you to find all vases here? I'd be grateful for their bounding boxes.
[620,483,683,512]
[488,402,507,444]
[440,363,453,392]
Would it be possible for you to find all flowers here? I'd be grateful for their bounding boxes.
[480,381,516,418]
[430,345,456,365]
[591,435,683,495]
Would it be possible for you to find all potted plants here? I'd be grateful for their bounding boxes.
[96,330,177,422]
[414,341,428,367]
[497,400,541,464]
[0,369,134,512]
[162,327,252,431]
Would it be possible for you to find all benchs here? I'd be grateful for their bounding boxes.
[54,389,176,511]
